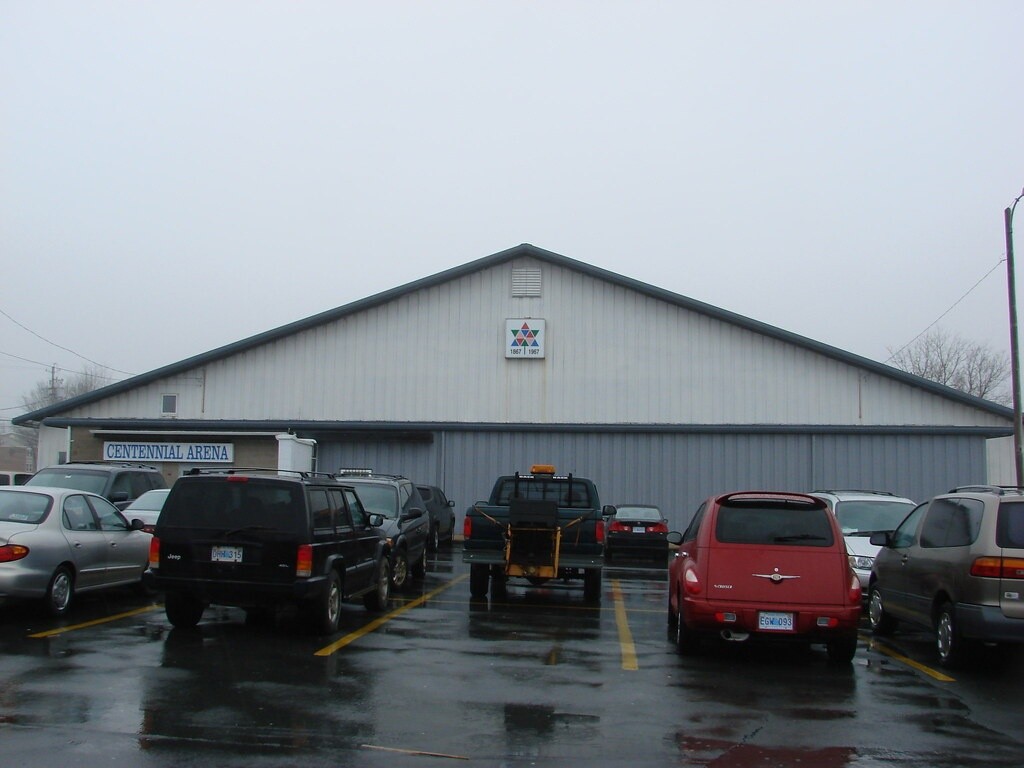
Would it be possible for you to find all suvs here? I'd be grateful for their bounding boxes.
[329,472,431,593]
[11,459,167,514]
[145,466,393,639]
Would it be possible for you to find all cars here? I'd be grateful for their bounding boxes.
[116,488,172,535]
[600,503,670,560]
[665,490,864,664]
[0,484,154,620]
[865,484,1024,671]
[783,489,920,601]
[412,484,456,554]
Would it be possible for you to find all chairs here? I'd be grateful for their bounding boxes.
[563,491,582,502]
[507,491,524,500]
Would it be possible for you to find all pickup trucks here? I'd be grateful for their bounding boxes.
[462,464,617,599]
